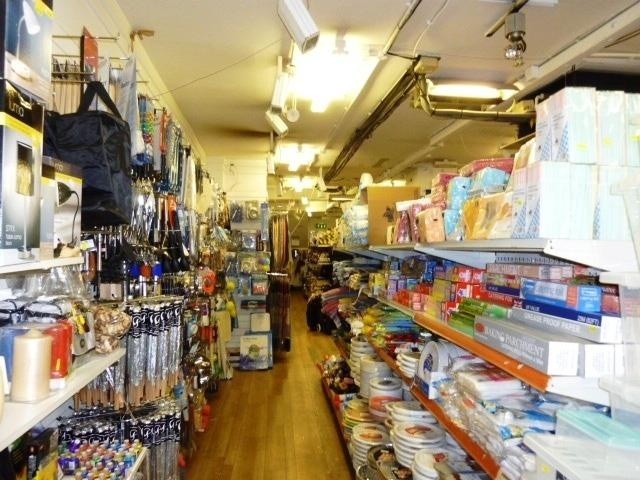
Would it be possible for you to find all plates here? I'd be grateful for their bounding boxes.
[342,337,473,479]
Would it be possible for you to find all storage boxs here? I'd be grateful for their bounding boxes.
[0,0,83,267]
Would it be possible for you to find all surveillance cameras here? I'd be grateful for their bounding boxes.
[502,42,529,64]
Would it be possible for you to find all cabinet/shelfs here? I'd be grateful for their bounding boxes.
[0,254,155,480]
[302,239,640,479]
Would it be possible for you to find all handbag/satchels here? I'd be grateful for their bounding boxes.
[43,82,132,230]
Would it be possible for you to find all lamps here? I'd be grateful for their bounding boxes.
[502,12,528,67]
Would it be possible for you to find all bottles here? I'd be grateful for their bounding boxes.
[56,438,144,479]
